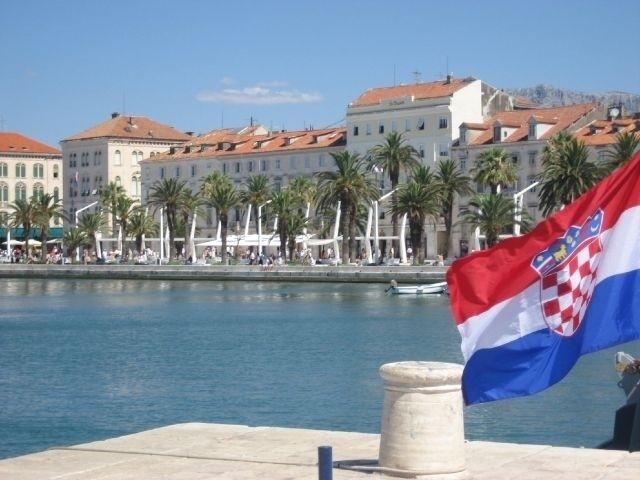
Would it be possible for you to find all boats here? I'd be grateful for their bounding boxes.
[390,277,448,295]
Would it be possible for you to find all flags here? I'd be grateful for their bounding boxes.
[445,150,640,406]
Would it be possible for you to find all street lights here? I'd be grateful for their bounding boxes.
[257,199,273,257]
